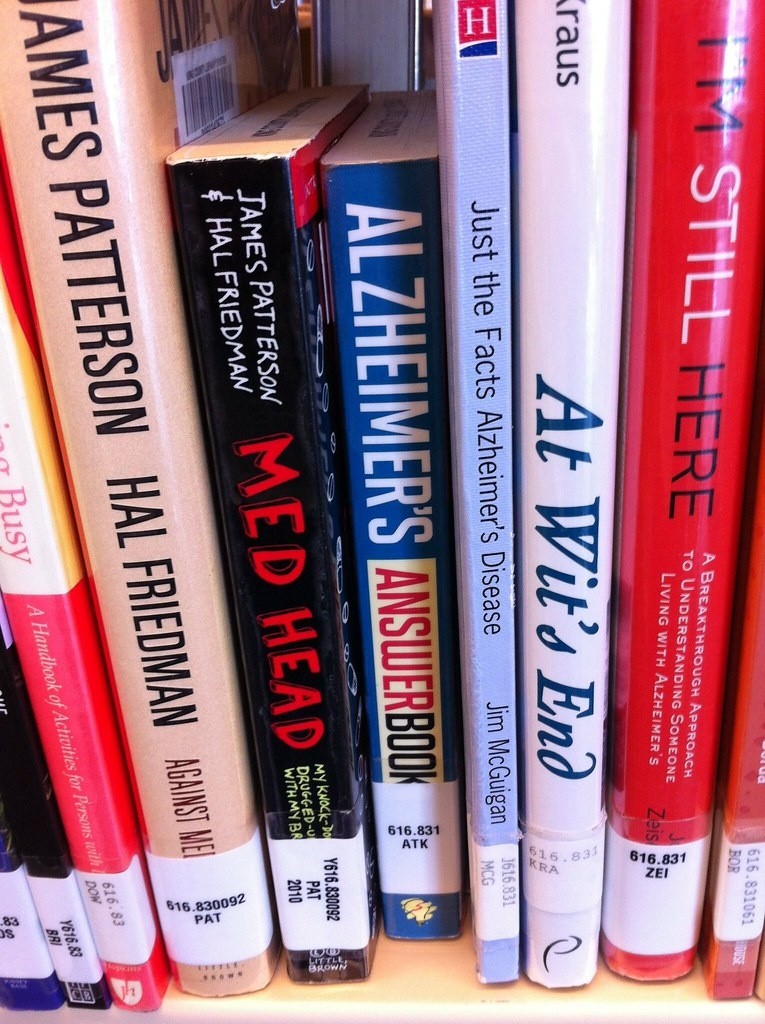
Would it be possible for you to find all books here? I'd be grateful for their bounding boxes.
[0,0,765,1014]
